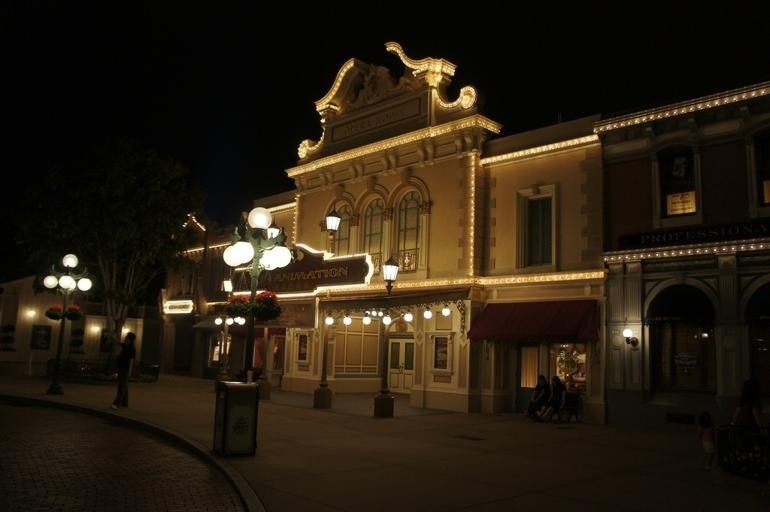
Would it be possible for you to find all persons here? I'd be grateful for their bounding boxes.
[108,330,137,411]
[725,378,764,429]
[525,374,551,421]
[535,375,568,422]
[689,411,721,474]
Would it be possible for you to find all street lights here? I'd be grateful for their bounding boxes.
[42,253,93,395]
[222,205,291,380]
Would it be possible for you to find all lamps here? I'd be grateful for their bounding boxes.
[267,215,281,238]
[319,204,342,232]
[623,328,638,347]
[382,255,399,294]
[324,303,451,326]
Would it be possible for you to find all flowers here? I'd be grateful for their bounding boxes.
[225,294,252,316]
[46,304,64,320]
[252,291,282,321]
[67,305,81,320]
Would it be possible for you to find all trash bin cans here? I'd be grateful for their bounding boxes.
[213,380,259,457]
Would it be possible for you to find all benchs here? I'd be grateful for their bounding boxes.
[529,392,579,424]
